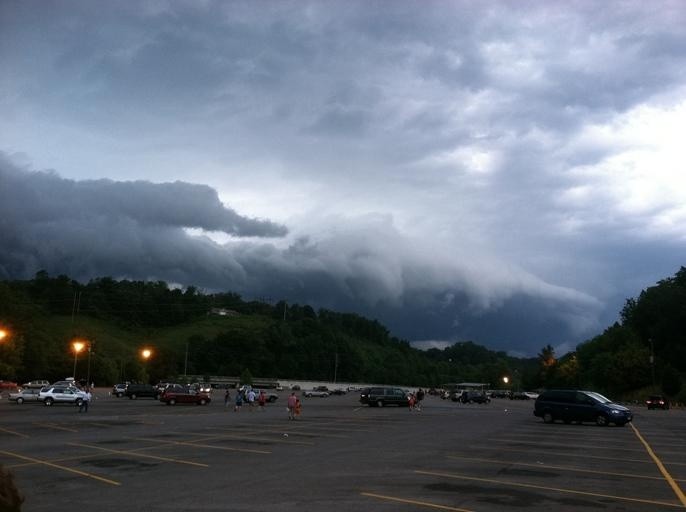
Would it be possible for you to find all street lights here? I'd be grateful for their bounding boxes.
[71,341,84,377]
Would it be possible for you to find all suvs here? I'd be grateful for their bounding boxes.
[113,384,162,400]
[359,387,417,407]
[533,390,633,427]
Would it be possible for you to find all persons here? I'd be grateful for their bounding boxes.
[287,391,296,421]
[77,389,91,413]
[0,464,25,512]
[296,398,302,417]
[407,388,424,412]
[223,385,267,412]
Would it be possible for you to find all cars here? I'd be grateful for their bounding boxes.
[160,382,211,405]
[491,391,539,400]
[304,390,329,398]
[8,379,91,407]
[647,395,669,410]
[244,389,278,403]
[440,390,491,405]
